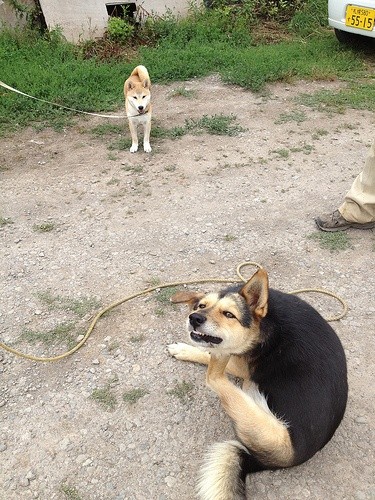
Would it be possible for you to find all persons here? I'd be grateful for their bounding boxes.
[315,144,375,232]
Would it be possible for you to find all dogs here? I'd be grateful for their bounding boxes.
[167,270,348,500]
[123,65,152,153]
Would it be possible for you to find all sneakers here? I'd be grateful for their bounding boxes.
[315,208,375,231]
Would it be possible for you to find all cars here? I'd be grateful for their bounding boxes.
[327,0,375,43]
